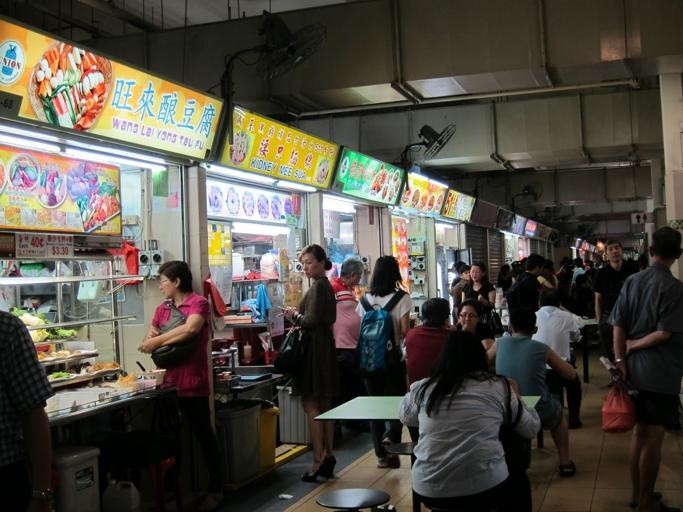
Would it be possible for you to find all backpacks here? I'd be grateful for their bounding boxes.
[358,290,407,373]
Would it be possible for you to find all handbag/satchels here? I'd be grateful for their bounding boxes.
[152,302,201,370]
[490,303,506,336]
[275,314,306,374]
[496,371,530,470]
[481,302,500,338]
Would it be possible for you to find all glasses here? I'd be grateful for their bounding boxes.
[159,279,172,286]
[459,313,480,319]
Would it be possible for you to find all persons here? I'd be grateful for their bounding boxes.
[559,256,603,292]
[138,261,225,512]
[275,244,337,481]
[594,239,648,378]
[0,312,56,512]
[532,290,585,430]
[506,254,545,307]
[607,226,683,512]
[402,297,457,392]
[451,264,471,320]
[446,262,465,326]
[537,259,559,288]
[486,308,576,477]
[399,330,540,512]
[456,298,495,351]
[462,261,496,309]
[498,264,515,297]
[355,255,412,469]
[332,259,363,451]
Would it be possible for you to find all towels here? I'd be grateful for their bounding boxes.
[256,283,271,319]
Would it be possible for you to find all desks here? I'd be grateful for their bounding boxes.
[215,373,285,400]
[581,318,600,382]
[313,394,543,512]
[42,374,176,444]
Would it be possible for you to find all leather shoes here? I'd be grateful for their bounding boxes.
[569,419,580,427]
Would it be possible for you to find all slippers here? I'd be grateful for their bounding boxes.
[629,492,663,505]
[636,504,678,512]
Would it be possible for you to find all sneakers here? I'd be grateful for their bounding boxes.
[382,436,400,469]
[377,457,386,467]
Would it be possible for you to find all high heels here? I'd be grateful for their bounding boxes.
[329,455,337,480]
[301,454,329,483]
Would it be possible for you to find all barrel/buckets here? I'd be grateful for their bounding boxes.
[102,480,140,512]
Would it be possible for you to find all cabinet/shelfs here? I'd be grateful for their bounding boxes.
[0,250,146,395]
[222,232,288,331]
[14,257,80,327]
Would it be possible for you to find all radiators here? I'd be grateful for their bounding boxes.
[276,385,314,445]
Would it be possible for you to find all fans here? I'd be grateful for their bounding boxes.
[511,180,545,217]
[400,121,458,191]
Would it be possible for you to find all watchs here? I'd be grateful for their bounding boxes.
[613,358,623,363]
[32,488,53,502]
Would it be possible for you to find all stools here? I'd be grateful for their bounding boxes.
[314,487,392,512]
[384,440,418,468]
[533,424,554,459]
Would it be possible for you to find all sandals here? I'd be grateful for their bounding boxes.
[164,491,173,498]
[559,459,575,478]
[195,491,227,511]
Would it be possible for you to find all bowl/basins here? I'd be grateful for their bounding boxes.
[241,359,254,364]
[137,368,167,386]
